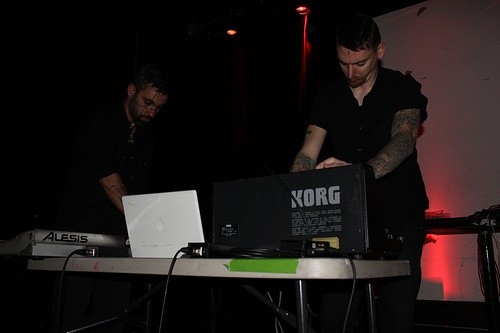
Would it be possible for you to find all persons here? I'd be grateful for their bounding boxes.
[49,66,170,333]
[291,16,428,333]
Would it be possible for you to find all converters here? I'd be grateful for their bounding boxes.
[277,240,330,260]
[187,242,238,259]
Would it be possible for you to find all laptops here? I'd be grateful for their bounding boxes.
[121,190,205,258]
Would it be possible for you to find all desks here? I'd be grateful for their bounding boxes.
[26,257,411,333]
